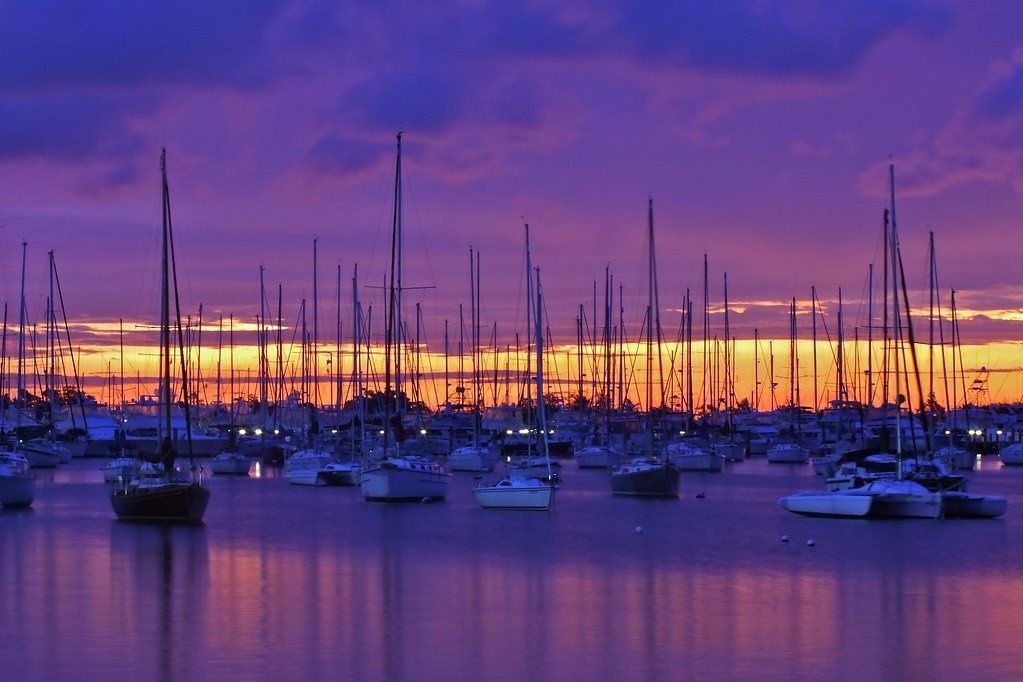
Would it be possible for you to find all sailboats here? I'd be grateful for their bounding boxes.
[0,128,1023,525]
